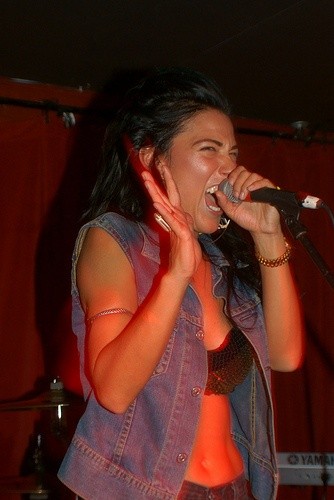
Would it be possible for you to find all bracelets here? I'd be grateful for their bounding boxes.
[254,237,291,268]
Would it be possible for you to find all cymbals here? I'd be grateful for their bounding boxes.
[0,473,67,494]
[0,390,89,411]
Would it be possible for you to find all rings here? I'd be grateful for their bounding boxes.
[153,211,175,232]
[275,185,282,190]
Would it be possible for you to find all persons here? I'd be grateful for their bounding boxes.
[57,68,303,500]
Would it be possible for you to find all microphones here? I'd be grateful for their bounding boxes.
[218,178,325,210]
